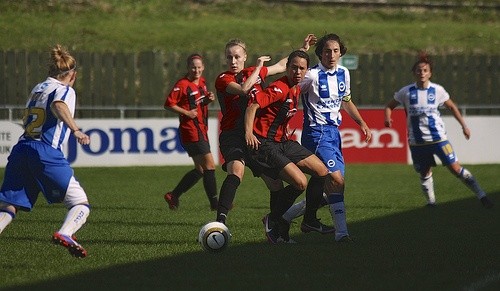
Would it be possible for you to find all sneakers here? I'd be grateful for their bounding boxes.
[262,213,280,245]
[333,232,351,243]
[51,231,87,259]
[164,191,180,210]
[300,218,335,234]
[210,200,234,213]
[278,216,299,243]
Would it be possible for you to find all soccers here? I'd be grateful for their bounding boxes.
[199,221,231,253]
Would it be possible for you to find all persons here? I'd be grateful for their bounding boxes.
[384,57,493,209]
[164,54,219,211]
[244,51,336,245]
[262,33,372,243]
[0,44,91,258]
[215,33,317,224]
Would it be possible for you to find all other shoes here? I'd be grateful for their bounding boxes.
[427,203,440,215]
[481,196,494,209]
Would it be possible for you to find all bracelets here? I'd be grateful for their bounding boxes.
[73,130,79,132]
